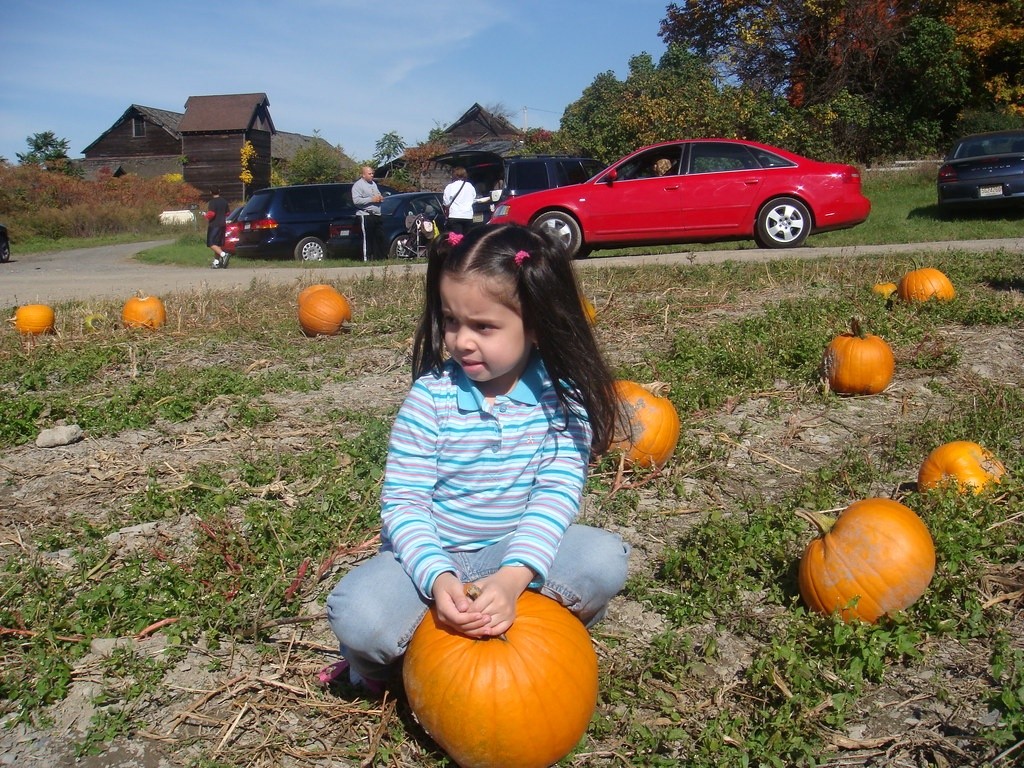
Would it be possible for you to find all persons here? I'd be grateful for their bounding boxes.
[652,156,672,177]
[443,165,477,236]
[316,223,630,698]
[350,167,385,262]
[200,185,230,269]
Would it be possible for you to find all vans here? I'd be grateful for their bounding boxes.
[235,183,402,262]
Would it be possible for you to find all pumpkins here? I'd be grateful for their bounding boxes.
[793,498,936,624]
[597,379,678,470]
[4,304,55,333]
[580,297,597,323]
[917,440,1007,500]
[123,289,168,326]
[403,582,599,768]
[298,284,357,335]
[873,258,954,303]
[822,316,893,394]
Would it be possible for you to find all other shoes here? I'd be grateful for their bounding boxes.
[320,660,383,695]
[211,260,223,269]
[222,252,231,268]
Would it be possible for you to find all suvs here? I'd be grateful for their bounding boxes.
[427,137,610,229]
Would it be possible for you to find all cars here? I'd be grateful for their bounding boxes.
[936,129,1023,215]
[221,206,244,254]
[329,192,445,260]
[0,224,11,262]
[489,137,872,260]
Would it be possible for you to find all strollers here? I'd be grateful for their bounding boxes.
[403,209,446,261]
